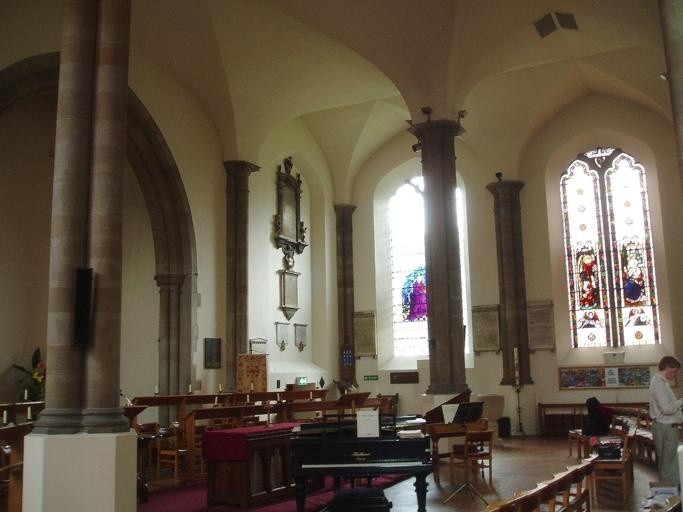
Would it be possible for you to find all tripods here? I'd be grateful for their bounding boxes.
[514,388,526,438]
[441,444,489,506]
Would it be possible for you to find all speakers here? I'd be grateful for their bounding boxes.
[497,417,511,438]
[73,267,94,344]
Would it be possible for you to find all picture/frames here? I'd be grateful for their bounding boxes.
[203,337,222,370]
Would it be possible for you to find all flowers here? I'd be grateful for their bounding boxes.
[11,348,46,400]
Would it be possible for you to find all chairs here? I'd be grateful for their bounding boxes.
[378,393,399,425]
[136,416,267,478]
[483,402,682,511]
[450,430,493,484]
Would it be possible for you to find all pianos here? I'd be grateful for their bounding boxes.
[289,416,436,512]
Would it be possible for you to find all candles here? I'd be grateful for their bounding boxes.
[154,384,347,406]
[26,406,32,418]
[3,409,9,424]
[24,389,28,399]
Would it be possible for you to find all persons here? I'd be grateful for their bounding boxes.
[649,356,683,492]
